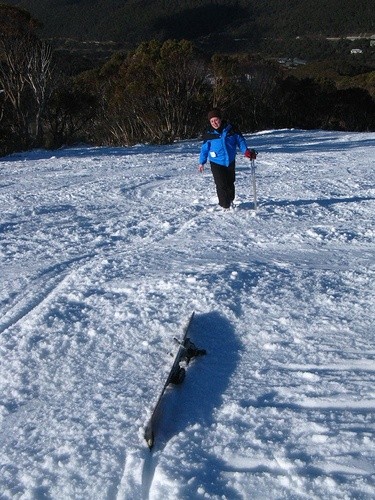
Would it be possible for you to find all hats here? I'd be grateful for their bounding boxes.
[208,109,221,121]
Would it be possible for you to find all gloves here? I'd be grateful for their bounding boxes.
[246,150,256,159]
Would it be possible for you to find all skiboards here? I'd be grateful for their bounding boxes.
[250,148,257,210]
[144,310,195,452]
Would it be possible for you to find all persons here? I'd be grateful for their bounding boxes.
[197,109,256,208]
